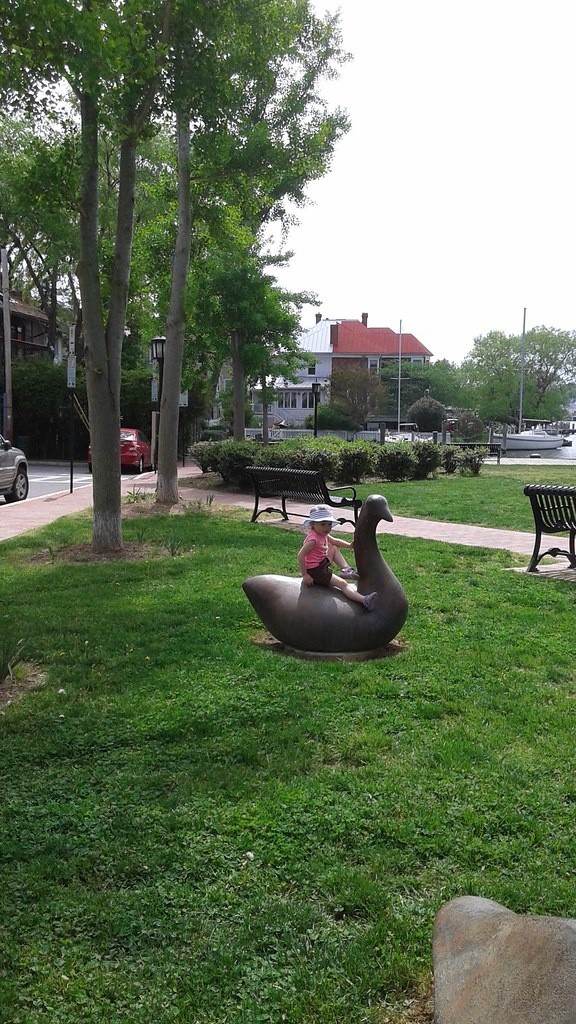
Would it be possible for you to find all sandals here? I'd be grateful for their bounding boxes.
[340,567,360,580]
[364,592,378,612]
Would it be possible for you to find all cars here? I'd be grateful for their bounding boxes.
[0,434,29,503]
[88,428,155,474]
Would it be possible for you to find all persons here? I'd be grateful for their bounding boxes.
[298,504,377,611]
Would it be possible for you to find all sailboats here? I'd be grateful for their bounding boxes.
[492,307,564,450]
[383,319,433,443]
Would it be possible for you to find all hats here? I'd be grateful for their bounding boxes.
[303,505,341,530]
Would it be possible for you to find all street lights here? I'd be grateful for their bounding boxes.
[312,378,321,438]
[151,331,166,419]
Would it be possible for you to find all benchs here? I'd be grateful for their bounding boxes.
[440,442,501,465]
[245,467,362,529]
[524,485,576,572]
